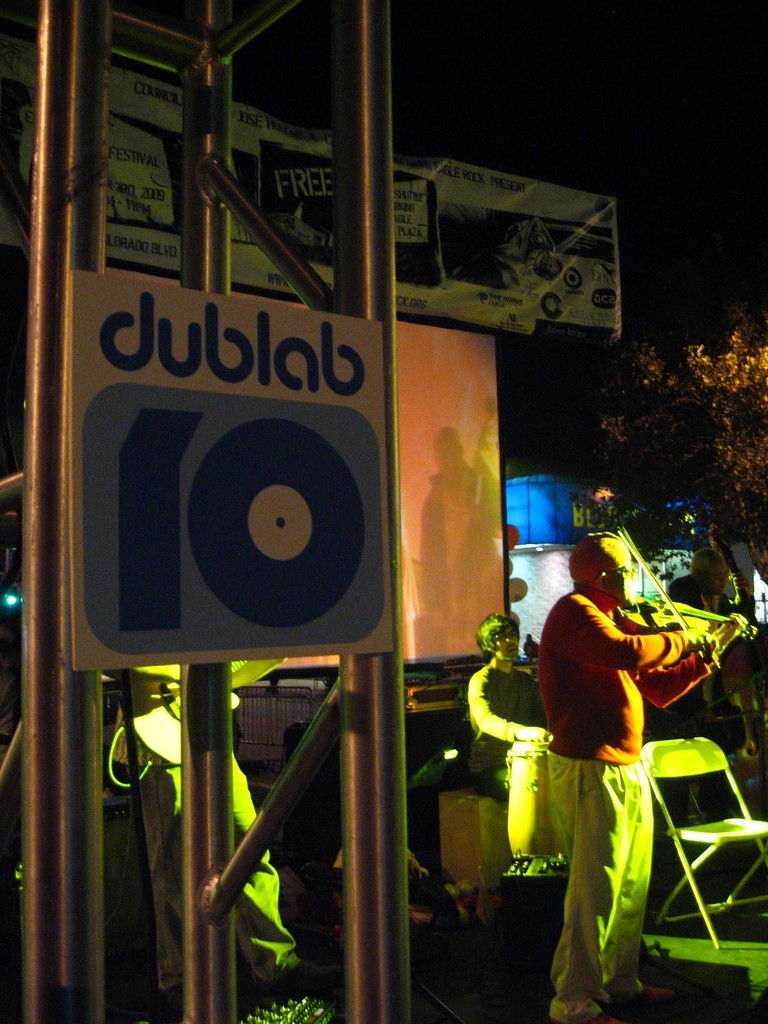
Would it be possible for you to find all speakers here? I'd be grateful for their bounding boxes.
[496,855,573,970]
[101,793,154,979]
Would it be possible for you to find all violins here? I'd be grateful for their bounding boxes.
[614,595,758,641]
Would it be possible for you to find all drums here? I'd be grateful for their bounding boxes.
[505,740,567,857]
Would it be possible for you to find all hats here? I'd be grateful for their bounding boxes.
[569,532,632,582]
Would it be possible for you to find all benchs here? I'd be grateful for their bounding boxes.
[438,789,512,893]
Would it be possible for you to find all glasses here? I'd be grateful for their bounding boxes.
[492,631,516,641]
[709,571,730,580]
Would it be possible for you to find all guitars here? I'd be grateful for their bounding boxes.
[122,648,293,770]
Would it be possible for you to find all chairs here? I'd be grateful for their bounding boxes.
[645,736,768,949]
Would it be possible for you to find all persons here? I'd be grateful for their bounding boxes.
[110,667,348,1014]
[464,530,768,1024]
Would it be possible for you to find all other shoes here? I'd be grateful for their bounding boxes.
[552,1011,628,1024]
[592,984,676,1009]
